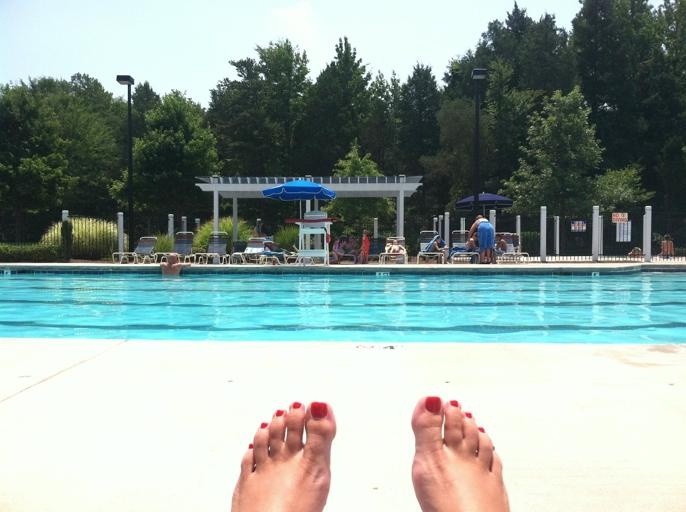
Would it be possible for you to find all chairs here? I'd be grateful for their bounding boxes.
[339,230,529,264]
[112,232,316,266]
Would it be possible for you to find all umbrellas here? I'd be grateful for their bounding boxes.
[455,191,515,216]
[260,177,337,219]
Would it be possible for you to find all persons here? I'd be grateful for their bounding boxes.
[159,252,191,277]
[330,235,349,264]
[657,234,676,260]
[263,238,282,265]
[385,239,409,264]
[468,213,496,264]
[254,221,262,237]
[360,229,371,264]
[466,231,508,264]
[629,247,644,258]
[228,394,511,510]
[427,236,450,264]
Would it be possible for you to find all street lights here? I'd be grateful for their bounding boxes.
[116,75,134,262]
[471,68,488,222]
